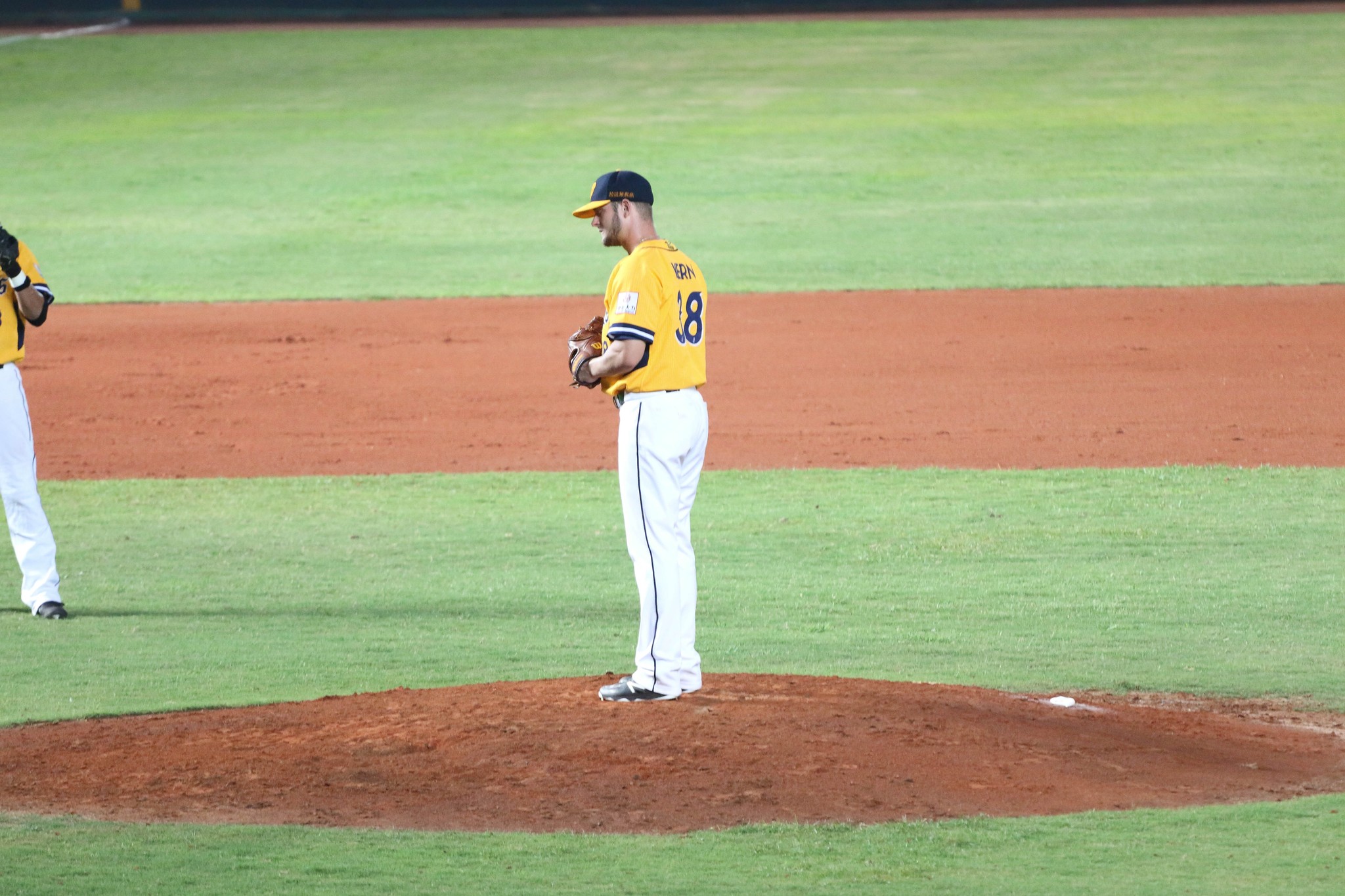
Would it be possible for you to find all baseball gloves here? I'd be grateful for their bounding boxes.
[568,316,604,389]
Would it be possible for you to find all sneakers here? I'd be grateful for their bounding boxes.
[37,601,68,621]
[599,680,680,702]
[619,676,696,693]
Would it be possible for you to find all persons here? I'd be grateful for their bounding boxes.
[566,171,710,708]
[0,224,68,619]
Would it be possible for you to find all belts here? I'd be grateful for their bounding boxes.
[613,388,680,409]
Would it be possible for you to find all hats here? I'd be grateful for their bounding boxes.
[572,169,654,219]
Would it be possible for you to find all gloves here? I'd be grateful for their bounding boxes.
[0,227,31,292]
